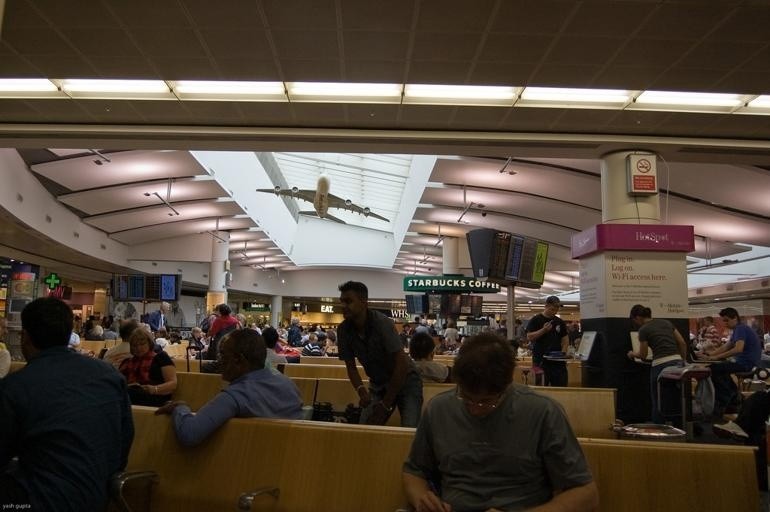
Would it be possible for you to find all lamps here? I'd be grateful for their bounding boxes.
[199,216,227,244]
[254,256,272,280]
[89,147,111,166]
[230,241,249,261]
[498,156,518,175]
[413,223,452,275]
[143,177,180,216]
[277,267,288,286]
[456,186,486,224]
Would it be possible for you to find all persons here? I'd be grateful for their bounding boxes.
[337,280,424,427]
[0,295,135,512]
[402,330,600,512]
[101,318,139,369]
[627,303,688,421]
[155,328,304,447]
[525,296,570,387]
[399,313,581,384]
[144,301,338,373]
[67,310,117,357]
[695,308,770,440]
[118,327,178,407]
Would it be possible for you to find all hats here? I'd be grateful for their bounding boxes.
[545,296,564,307]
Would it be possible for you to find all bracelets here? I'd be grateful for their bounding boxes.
[379,399,393,413]
[155,385,158,395]
[356,384,365,393]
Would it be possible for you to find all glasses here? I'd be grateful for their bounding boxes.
[338,297,356,305]
[454,384,508,410]
